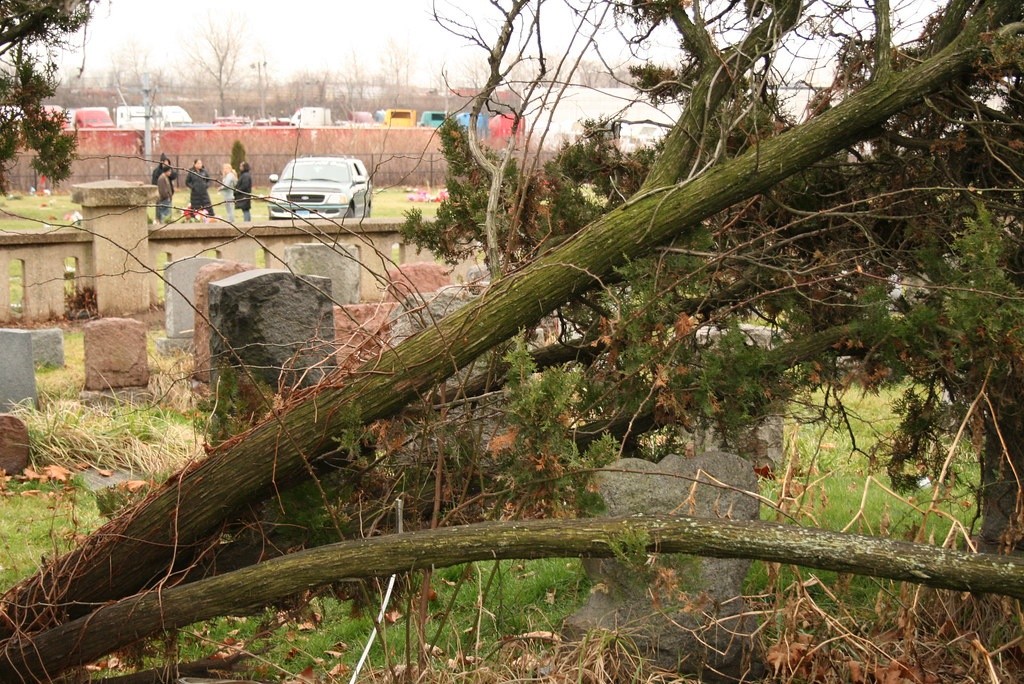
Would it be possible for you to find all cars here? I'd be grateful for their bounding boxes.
[268,157,372,221]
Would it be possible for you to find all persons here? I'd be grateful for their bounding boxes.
[157,166,172,220]
[153,154,175,197]
[217,164,238,222]
[233,161,252,219]
[186,158,215,217]
